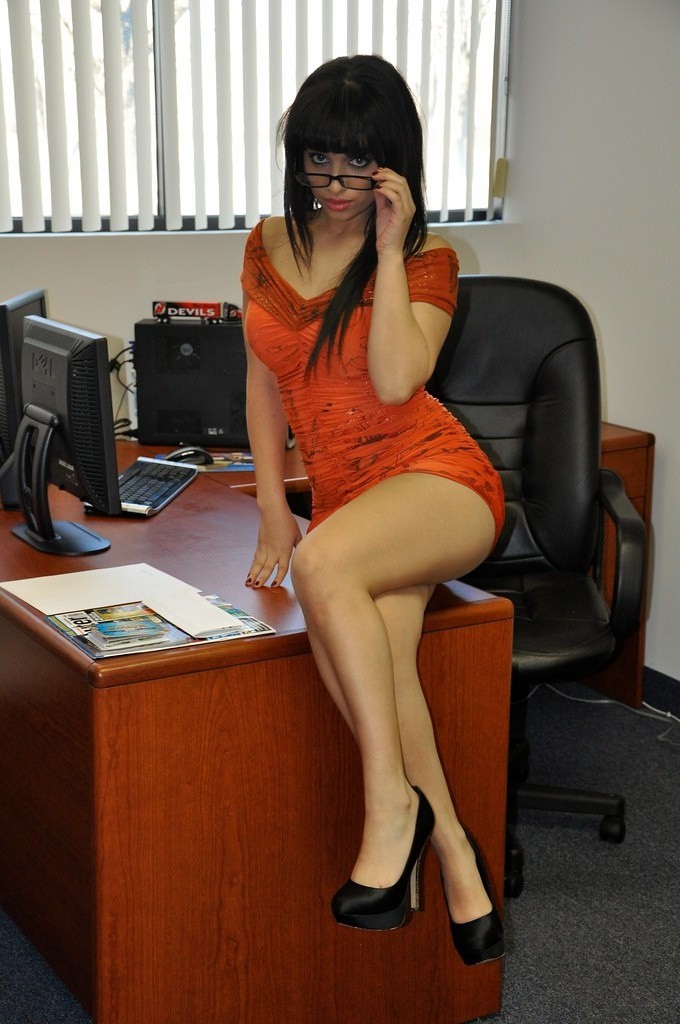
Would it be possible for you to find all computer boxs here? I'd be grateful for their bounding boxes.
[134,318,251,449]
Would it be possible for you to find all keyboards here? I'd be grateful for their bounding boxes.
[85,456,200,516]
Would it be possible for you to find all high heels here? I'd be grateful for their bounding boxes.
[440,826,505,966]
[331,786,435,932]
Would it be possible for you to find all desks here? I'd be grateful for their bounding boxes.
[134,420,655,707]
[0,439,513,1024]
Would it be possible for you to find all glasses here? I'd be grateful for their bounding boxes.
[293,157,378,191]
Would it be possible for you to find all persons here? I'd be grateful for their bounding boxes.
[243,54,512,966]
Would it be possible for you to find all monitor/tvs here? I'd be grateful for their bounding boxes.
[0,289,49,511]
[11,316,122,557]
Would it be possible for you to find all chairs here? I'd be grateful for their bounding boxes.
[423,274,647,899]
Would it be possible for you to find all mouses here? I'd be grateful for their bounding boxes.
[164,446,213,465]
